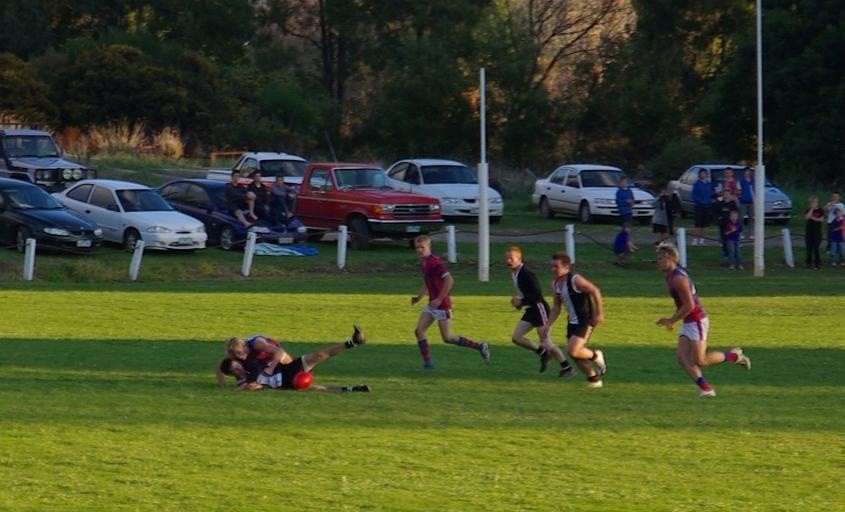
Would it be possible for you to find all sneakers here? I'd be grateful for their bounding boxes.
[480,342,491,361]
[557,366,577,378]
[729,264,744,271]
[732,346,752,372]
[583,378,603,389]
[539,350,552,373]
[423,361,435,370]
[353,383,371,394]
[721,235,756,248]
[806,261,845,270]
[655,237,707,249]
[699,388,716,400]
[593,349,607,374]
[352,323,366,347]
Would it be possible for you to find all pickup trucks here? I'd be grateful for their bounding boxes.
[247,156,448,248]
[199,149,332,195]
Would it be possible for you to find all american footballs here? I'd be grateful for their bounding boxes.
[293,372,312,388]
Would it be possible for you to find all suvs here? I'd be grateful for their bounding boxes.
[0,121,96,192]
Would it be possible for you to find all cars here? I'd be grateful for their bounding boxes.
[155,174,308,254]
[528,161,658,225]
[0,175,107,257]
[47,174,212,257]
[665,161,797,226]
[373,152,506,226]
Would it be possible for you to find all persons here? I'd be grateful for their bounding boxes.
[216,336,292,387]
[247,170,269,217]
[225,168,258,227]
[539,251,608,388]
[218,323,372,394]
[409,234,490,375]
[268,175,297,222]
[608,155,844,274]
[503,245,574,376]
[651,242,752,397]
